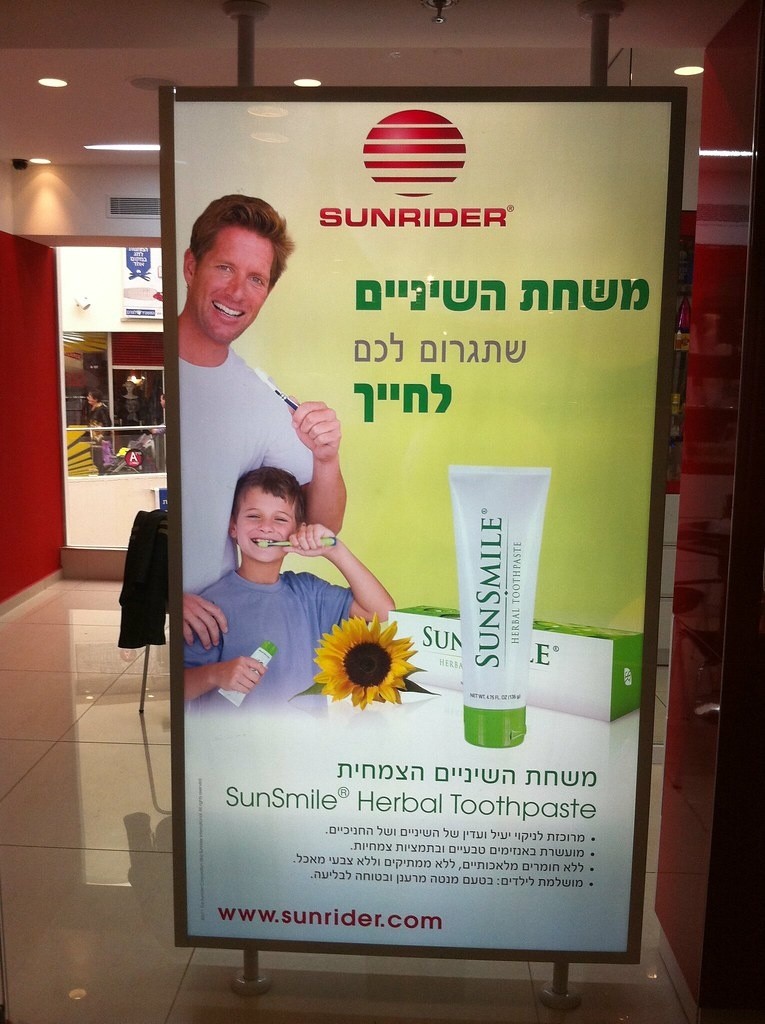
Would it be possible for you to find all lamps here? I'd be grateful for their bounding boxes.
[131,370,142,384]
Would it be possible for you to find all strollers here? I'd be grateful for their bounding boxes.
[91,427,158,476]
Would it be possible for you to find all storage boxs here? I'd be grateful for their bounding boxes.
[387,602,642,722]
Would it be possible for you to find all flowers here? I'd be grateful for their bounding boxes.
[285,611,443,712]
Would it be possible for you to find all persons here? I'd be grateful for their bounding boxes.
[116,382,147,437]
[183,466,397,712]
[149,394,167,437]
[83,389,114,476]
[176,192,349,651]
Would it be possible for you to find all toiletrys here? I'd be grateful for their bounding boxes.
[448,466,551,748]
[219,639,279,708]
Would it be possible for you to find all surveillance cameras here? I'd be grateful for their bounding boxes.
[12,159,28,170]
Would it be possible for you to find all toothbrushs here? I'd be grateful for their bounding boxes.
[257,539,338,550]
[254,366,301,411]
